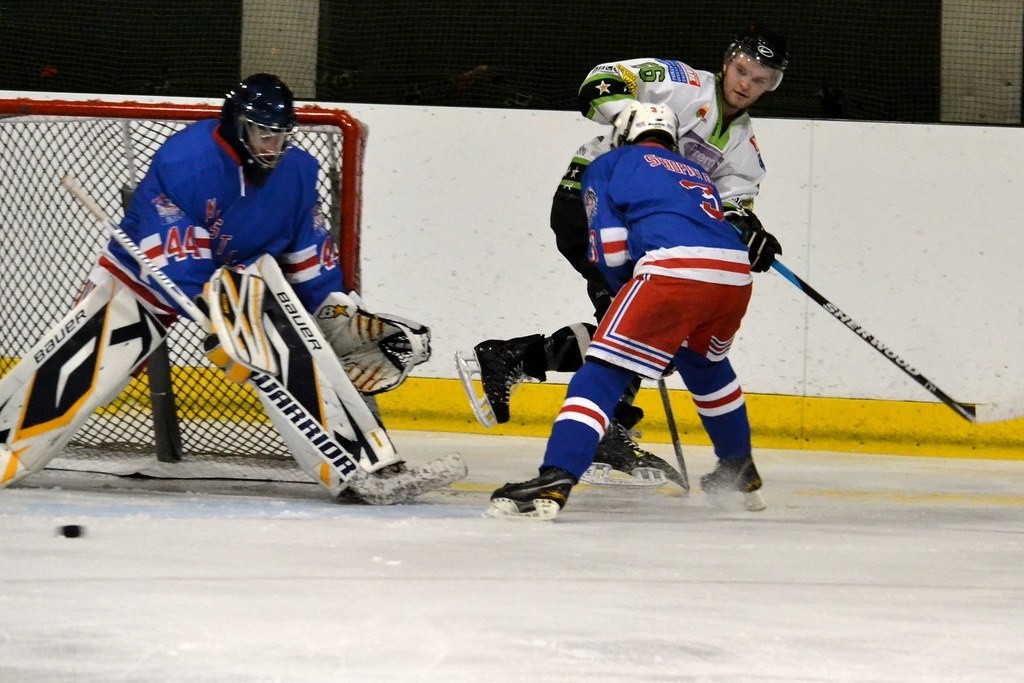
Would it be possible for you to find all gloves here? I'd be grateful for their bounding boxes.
[728,210,782,274]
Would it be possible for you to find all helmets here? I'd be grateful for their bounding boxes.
[607,104,679,152]
[723,24,792,79]
[221,73,297,187]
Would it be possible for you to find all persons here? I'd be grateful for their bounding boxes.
[0,73,431,505]
[474,25,789,521]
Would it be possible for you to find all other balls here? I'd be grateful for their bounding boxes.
[59,524,85,538]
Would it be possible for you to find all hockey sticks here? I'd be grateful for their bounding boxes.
[59,175,471,507]
[731,223,1023,423]
[658,378,691,492]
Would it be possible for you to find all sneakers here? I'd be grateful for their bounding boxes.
[579,402,668,485]
[455,333,547,428]
[485,466,577,521]
[701,458,765,512]
